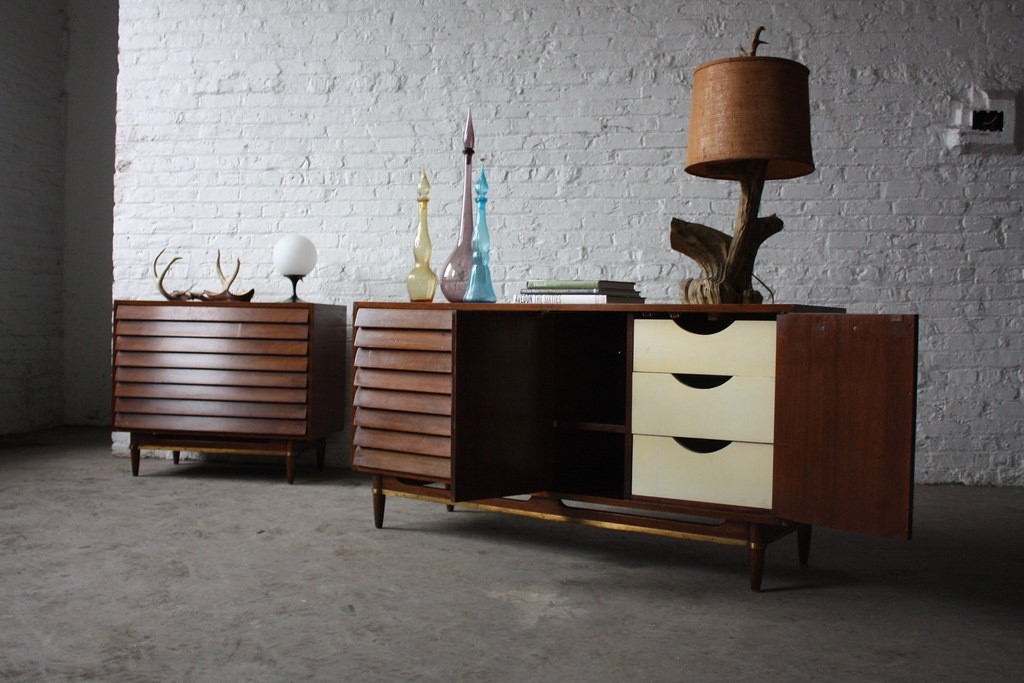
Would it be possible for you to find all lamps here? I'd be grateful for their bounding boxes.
[273,234,317,303]
[667,25,817,306]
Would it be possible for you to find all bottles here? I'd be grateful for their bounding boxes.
[406,173,438,301]
[462,165,496,303]
[440,109,475,302]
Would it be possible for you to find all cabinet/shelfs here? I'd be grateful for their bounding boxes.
[349,302,920,591]
[111,299,347,484]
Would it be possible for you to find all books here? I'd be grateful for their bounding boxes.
[518,279,648,304]
